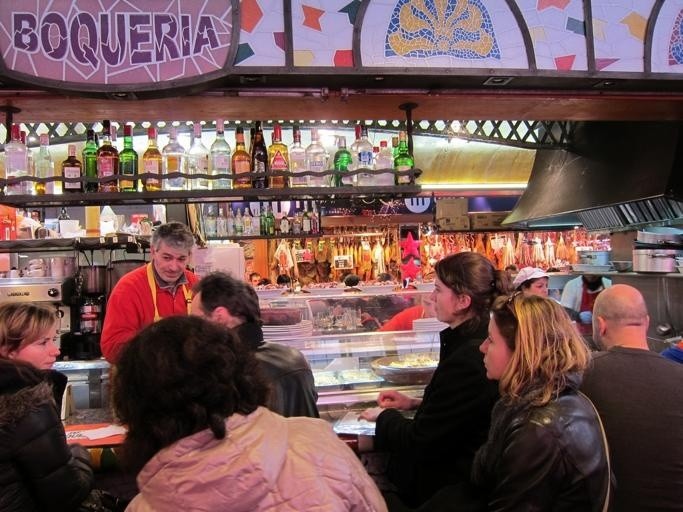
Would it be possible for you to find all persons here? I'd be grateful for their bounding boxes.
[250,273,262,287]
[581,285,683,512]
[188,274,319,418]
[325,276,375,316]
[561,269,613,341]
[369,272,408,315]
[473,294,615,512]
[356,253,510,512]
[376,305,424,331]
[513,266,550,295]
[113,315,388,510]
[1,303,94,512]
[100,222,201,367]
[277,274,290,285]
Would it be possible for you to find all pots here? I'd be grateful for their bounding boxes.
[632,226,683,273]
[577,251,609,264]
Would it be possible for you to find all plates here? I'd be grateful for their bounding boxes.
[412,318,446,330]
[262,320,313,340]
[257,288,289,296]
[353,283,403,294]
[342,368,385,389]
[313,369,342,391]
[413,282,437,291]
[301,288,350,295]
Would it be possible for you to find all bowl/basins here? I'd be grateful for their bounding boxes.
[675,255,683,273]
[571,264,611,272]
[372,352,440,384]
[609,261,632,271]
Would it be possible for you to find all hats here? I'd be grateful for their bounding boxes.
[513,266,550,289]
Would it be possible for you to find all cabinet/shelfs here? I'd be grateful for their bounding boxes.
[1,92,426,211]
[256,283,449,488]
[50,361,129,470]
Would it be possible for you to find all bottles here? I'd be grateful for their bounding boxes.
[251,120,268,189]
[95,134,100,149]
[242,207,252,236]
[351,125,361,187]
[141,127,162,192]
[287,125,304,150]
[162,128,187,191]
[216,207,226,237]
[260,209,266,236]
[311,212,319,235]
[34,134,54,195]
[252,209,260,236]
[305,128,327,187]
[266,209,274,236]
[119,125,138,192]
[226,205,235,236]
[372,146,379,165]
[205,205,216,238]
[374,141,394,186]
[235,206,242,236]
[292,212,301,236]
[394,131,414,185]
[96,119,118,193]
[61,144,83,193]
[267,124,288,190]
[187,123,210,190]
[356,127,373,186]
[82,129,98,192]
[248,128,256,154]
[20,130,33,195]
[280,212,290,236]
[4,125,28,196]
[302,211,311,236]
[391,137,398,158]
[231,127,252,190]
[210,118,231,190]
[112,126,118,150]
[289,131,308,187]
[333,139,353,187]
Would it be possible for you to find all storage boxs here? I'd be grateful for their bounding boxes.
[472,211,510,229]
[435,197,467,215]
[437,215,472,230]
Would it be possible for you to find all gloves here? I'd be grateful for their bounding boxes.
[579,311,592,324]
